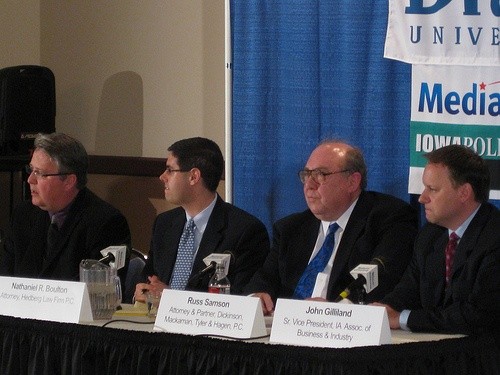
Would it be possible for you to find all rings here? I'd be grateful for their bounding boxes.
[155,292,158,298]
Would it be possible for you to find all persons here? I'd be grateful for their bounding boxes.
[241,142,417,315]
[0,132,132,285]
[367,144,500,334]
[123,137,269,311]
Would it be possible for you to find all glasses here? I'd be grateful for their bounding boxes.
[165,167,190,174]
[298,168,353,184]
[24,164,73,178]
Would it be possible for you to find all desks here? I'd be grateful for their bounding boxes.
[0,306,469,375]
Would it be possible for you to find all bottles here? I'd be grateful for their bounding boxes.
[208,259,231,294]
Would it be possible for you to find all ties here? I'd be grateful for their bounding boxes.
[444,231,460,304]
[290,222,340,300]
[43,215,59,262]
[168,216,197,290]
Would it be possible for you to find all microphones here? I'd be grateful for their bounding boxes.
[334,255,389,302]
[98,244,131,271]
[192,250,235,281]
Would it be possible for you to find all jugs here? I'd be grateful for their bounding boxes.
[79,260,122,320]
